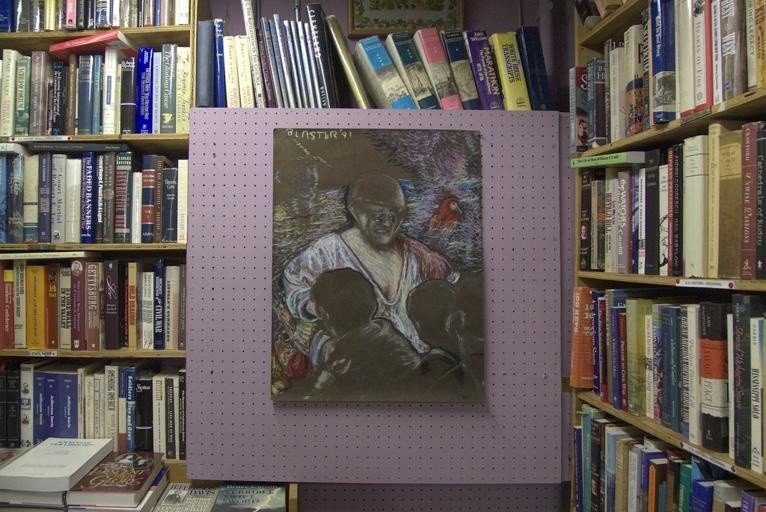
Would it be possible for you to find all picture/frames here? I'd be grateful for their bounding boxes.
[348,0,464,39]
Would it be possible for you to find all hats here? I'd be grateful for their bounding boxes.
[347,175,406,217]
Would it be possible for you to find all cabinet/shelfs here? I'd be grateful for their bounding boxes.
[0,0,213,488]
[570,0,766,512]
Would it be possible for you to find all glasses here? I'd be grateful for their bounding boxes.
[370,213,395,223]
